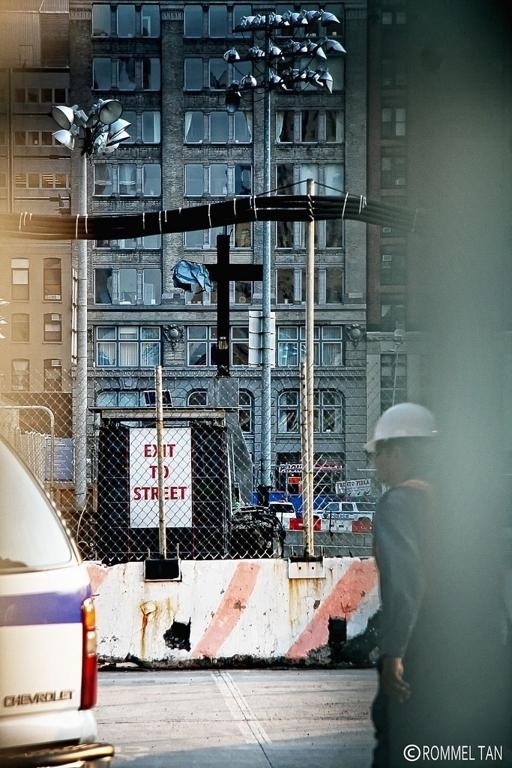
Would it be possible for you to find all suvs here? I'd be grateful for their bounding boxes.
[0,434,123,768]
[310,497,376,533]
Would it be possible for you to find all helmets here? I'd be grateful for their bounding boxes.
[360,403,442,454]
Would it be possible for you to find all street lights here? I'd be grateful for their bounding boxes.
[219,9,346,505]
[51,92,131,506]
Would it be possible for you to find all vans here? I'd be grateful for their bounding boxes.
[267,499,298,531]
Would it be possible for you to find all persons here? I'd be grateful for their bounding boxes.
[364,403,439,768]
